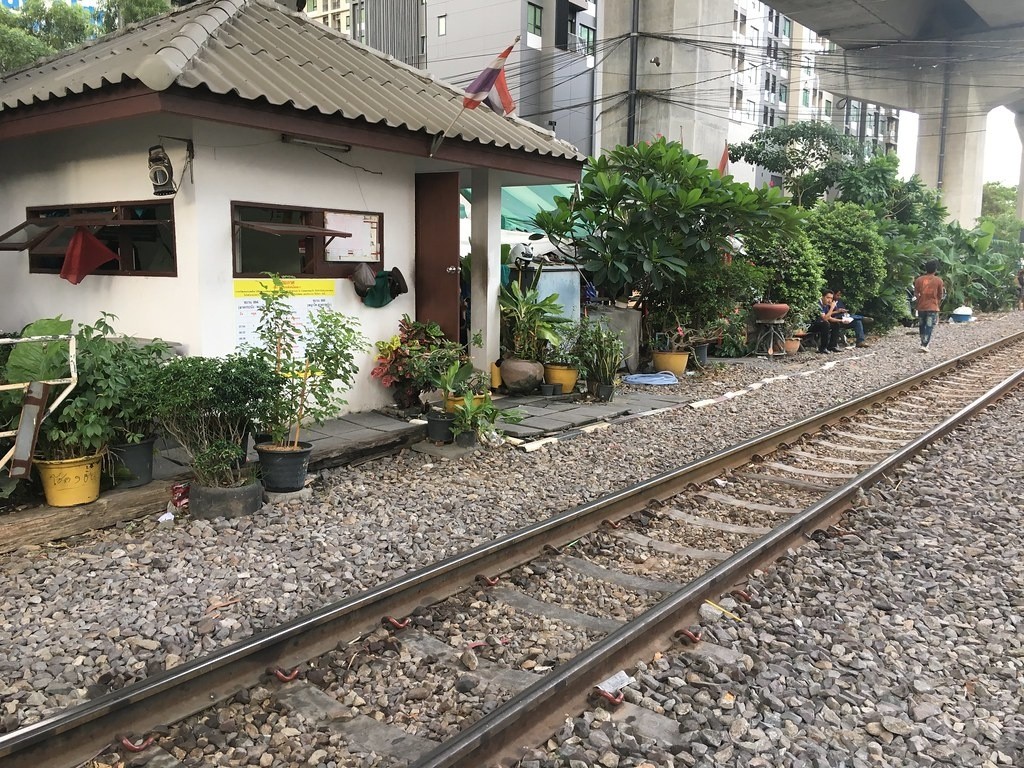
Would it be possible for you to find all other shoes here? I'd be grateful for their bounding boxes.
[828,347,842,352]
[819,348,830,354]
[918,346,930,353]
[856,342,869,348]
[863,316,874,325]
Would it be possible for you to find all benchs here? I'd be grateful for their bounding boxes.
[793,329,850,351]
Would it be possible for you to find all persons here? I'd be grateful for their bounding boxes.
[915,261,943,352]
[807,288,874,354]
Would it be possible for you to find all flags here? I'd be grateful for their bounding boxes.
[461,45,516,118]
[718,145,728,177]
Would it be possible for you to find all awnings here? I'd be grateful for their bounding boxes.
[460,166,602,240]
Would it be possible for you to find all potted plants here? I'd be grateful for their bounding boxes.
[29,134,828,522]
